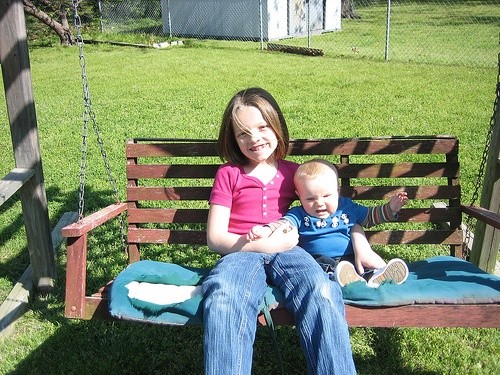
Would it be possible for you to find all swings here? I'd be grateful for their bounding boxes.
[61,0,500,329]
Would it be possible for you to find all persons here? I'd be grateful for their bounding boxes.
[202,88,387,375]
[246,159,410,289]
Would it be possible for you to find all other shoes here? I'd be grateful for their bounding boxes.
[334,261,367,287]
[368,258,409,289]
